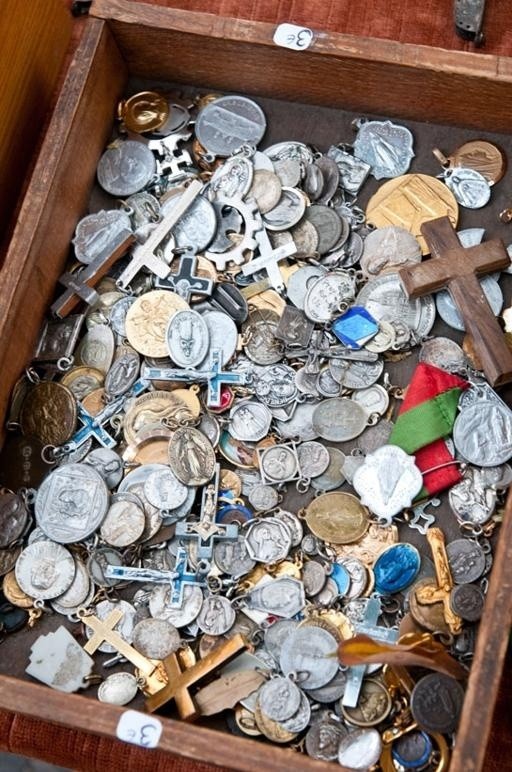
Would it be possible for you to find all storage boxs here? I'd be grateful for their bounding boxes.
[0,0,511,771]
